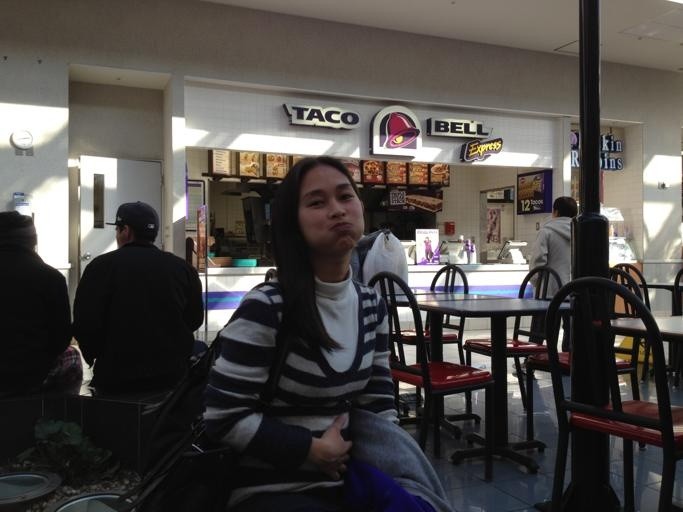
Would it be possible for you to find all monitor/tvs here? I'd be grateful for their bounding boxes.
[498,240,528,261]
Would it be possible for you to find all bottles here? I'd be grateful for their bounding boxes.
[458,235,477,265]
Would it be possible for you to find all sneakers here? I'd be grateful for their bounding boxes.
[512,359,535,375]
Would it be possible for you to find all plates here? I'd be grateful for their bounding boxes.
[431,164,449,175]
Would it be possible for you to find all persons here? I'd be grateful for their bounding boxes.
[203,152,457,511]
[1,210,72,397]
[422,237,435,261]
[71,202,204,398]
[513,196,578,380]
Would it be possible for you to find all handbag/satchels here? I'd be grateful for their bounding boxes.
[41,345,83,396]
[115,346,250,512]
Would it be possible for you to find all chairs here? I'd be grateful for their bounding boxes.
[461,264,566,417]
[541,274,682,511]
[382,263,473,415]
[368,269,500,483]
[523,260,682,455]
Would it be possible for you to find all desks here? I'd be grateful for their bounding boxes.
[405,294,579,480]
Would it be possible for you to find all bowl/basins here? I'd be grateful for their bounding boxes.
[208,257,257,268]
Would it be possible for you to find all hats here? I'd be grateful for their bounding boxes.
[0,211,36,238]
[105,201,159,238]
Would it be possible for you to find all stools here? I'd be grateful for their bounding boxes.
[0,379,179,480]
